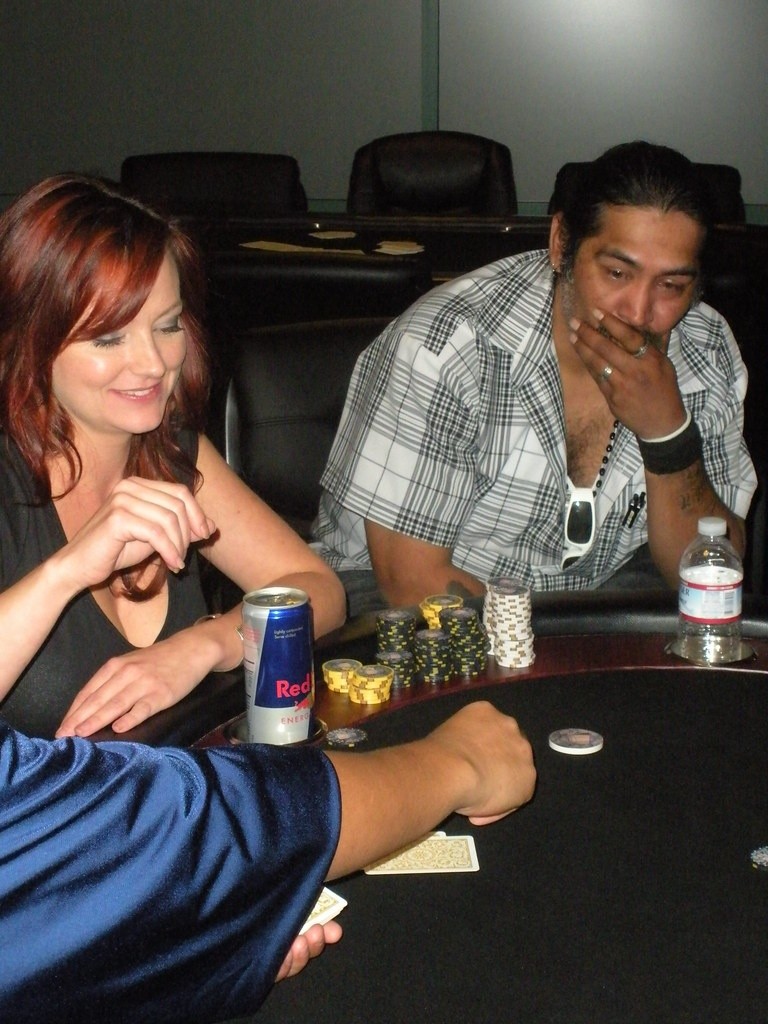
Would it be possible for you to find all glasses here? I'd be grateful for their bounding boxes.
[560,487,596,571]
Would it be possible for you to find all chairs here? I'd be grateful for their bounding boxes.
[548,161,743,202]
[120,151,310,198]
[223,315,398,540]
[347,130,517,201]
[205,251,431,318]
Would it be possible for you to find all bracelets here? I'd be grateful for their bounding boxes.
[636,407,702,475]
[193,612,245,672]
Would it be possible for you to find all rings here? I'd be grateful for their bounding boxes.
[596,364,613,382]
[632,339,650,359]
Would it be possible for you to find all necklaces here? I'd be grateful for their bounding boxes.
[566,418,620,498]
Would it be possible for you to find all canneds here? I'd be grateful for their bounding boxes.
[239,587,317,746]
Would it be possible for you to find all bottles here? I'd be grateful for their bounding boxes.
[679,517,745,661]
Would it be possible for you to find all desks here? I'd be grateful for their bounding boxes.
[83,588,768,1024]
[159,200,768,287]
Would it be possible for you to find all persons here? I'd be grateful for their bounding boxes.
[307,141,758,619]
[1,171,346,742]
[1,699,537,1024]
